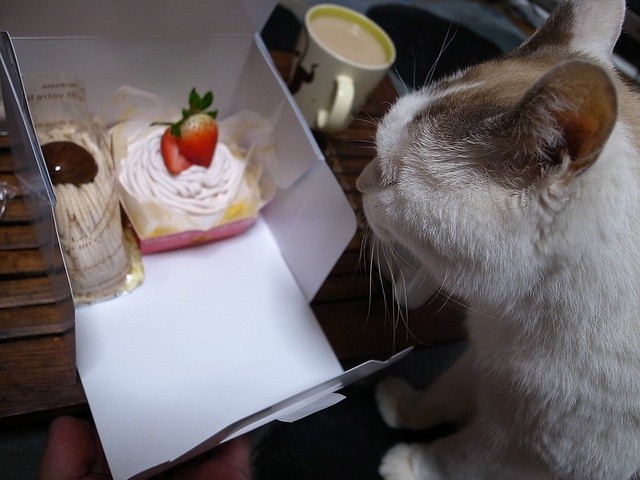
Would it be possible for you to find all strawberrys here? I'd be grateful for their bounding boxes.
[159,113,218,176]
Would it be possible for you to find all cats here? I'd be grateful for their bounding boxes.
[356,0,640,480]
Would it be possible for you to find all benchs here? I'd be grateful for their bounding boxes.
[0,50,465,433]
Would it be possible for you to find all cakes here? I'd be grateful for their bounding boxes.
[103,84,259,241]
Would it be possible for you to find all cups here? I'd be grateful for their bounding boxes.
[285,4,396,135]
